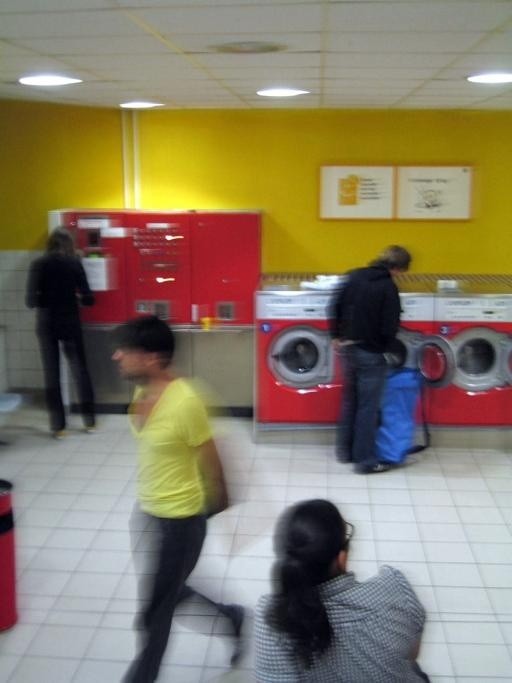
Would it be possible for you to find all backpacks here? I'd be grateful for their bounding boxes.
[372,368,429,463]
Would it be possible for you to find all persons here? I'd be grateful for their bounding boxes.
[251,499,429,683]
[326,244,410,474]
[26,228,95,437]
[111,314,244,682]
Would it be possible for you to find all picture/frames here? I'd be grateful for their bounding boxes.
[394,161,475,223]
[314,160,394,224]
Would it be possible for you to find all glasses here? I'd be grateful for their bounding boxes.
[338,520,355,553]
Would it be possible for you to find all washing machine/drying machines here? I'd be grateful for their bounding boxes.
[345,291,433,423]
[432,293,512,428]
[254,274,343,425]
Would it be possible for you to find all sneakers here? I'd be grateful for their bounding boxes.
[53,424,97,440]
[355,459,392,477]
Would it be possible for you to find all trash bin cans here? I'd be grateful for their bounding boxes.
[0,479,17,631]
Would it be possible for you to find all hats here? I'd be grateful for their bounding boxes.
[108,314,176,363]
